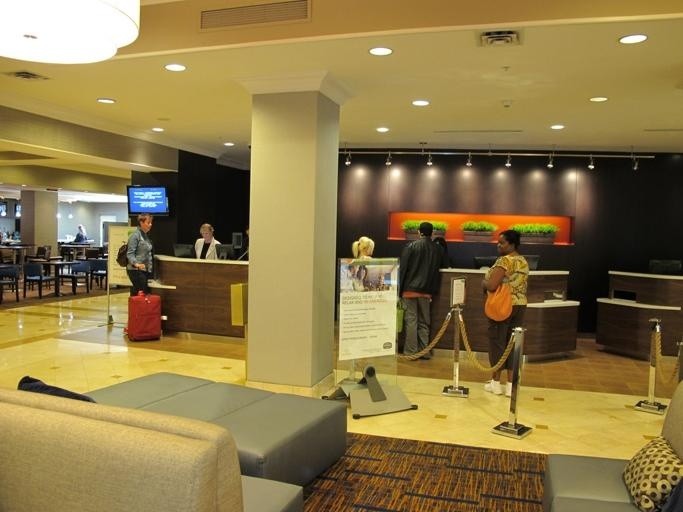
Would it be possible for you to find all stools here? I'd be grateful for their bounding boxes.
[83,372,347,486]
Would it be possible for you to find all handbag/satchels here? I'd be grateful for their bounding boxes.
[117,245,128,266]
[485,283,512,321]
[396,300,404,332]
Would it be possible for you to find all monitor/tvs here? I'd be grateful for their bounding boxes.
[126,183,170,217]
[215,244,238,260]
[174,244,196,259]
[472,256,498,269]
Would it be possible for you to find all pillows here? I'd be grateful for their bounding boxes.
[18,376,95,402]
[623,438,683,512]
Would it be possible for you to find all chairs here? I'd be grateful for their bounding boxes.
[0,240,108,303]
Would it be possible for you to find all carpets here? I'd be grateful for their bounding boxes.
[304,432,546,512]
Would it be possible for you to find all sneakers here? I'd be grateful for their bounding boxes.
[505,382,512,397]
[484,380,503,395]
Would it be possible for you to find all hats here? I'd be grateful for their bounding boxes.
[417,222,432,230]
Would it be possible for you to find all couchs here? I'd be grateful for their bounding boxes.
[546,382,683,512]
[1,387,304,512]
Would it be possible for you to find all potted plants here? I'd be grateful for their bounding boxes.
[401,220,447,240]
[512,224,558,244]
[462,222,496,241]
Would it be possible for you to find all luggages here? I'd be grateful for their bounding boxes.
[128,265,161,341]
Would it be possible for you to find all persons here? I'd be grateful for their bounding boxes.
[352,266,373,292]
[352,236,375,260]
[398,221,441,361]
[192,223,222,259]
[432,236,451,268]
[74,225,88,255]
[126,213,154,295]
[480,229,530,398]
